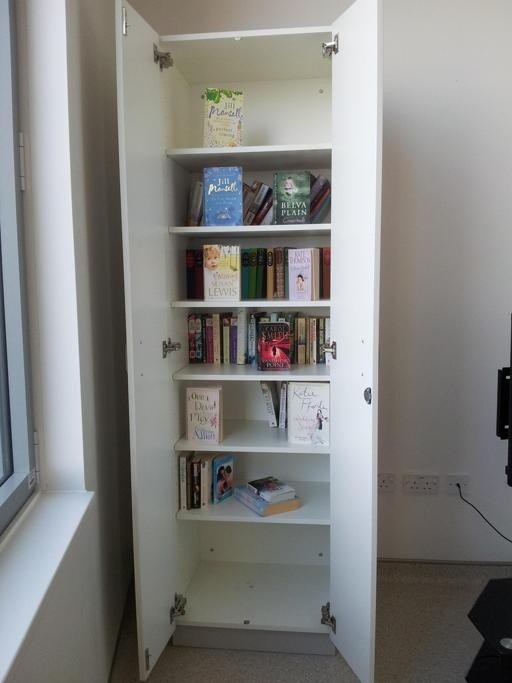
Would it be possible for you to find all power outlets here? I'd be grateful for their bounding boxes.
[403,474,437,496]
[379,473,398,492]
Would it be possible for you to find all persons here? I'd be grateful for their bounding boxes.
[298,275,304,292]
[203,247,221,292]
[216,465,232,495]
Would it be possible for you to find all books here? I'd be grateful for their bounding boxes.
[274,170,311,224]
[179,452,216,511]
[202,166,243,227]
[239,245,288,301]
[200,87,246,148]
[261,381,277,427]
[233,476,302,517]
[185,248,204,300]
[278,382,287,429]
[310,171,331,224]
[212,454,235,505]
[319,244,330,301]
[287,248,320,302]
[203,245,242,303]
[243,180,275,226]
[184,386,223,444]
[286,382,330,448]
[186,180,203,227]
[188,308,331,372]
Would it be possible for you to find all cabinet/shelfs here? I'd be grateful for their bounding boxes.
[116,0,382,683]
